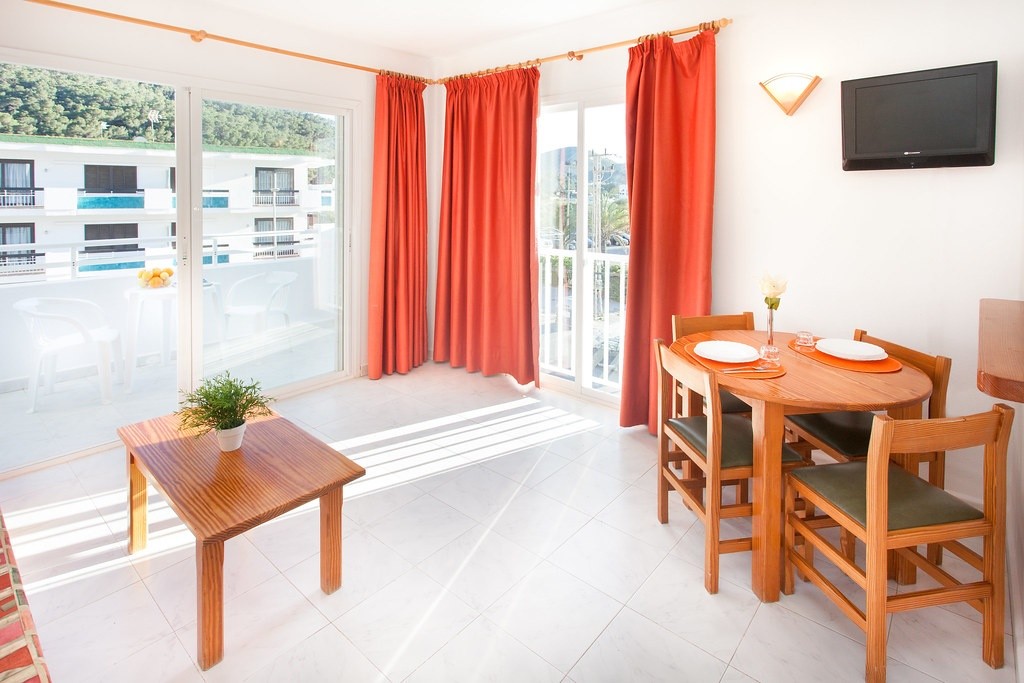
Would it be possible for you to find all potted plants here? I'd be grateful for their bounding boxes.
[172,368,279,452]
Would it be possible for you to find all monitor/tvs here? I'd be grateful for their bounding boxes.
[842,61,998,160]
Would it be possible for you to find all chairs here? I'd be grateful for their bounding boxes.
[652,337,817,596]
[781,327,953,568]
[778,402,1017,683]
[671,311,757,469]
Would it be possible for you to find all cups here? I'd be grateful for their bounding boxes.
[760,346,781,369]
[794,331,815,353]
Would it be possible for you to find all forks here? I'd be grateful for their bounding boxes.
[722,365,769,371]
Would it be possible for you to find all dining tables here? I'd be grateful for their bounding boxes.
[666,329,936,587]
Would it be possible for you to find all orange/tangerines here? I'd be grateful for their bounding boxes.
[137,267,174,289]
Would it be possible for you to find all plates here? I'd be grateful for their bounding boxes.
[694,341,760,363]
[815,338,889,361]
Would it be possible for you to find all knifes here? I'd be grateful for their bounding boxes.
[724,370,779,373]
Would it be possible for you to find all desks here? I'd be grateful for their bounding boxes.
[115,395,366,672]
